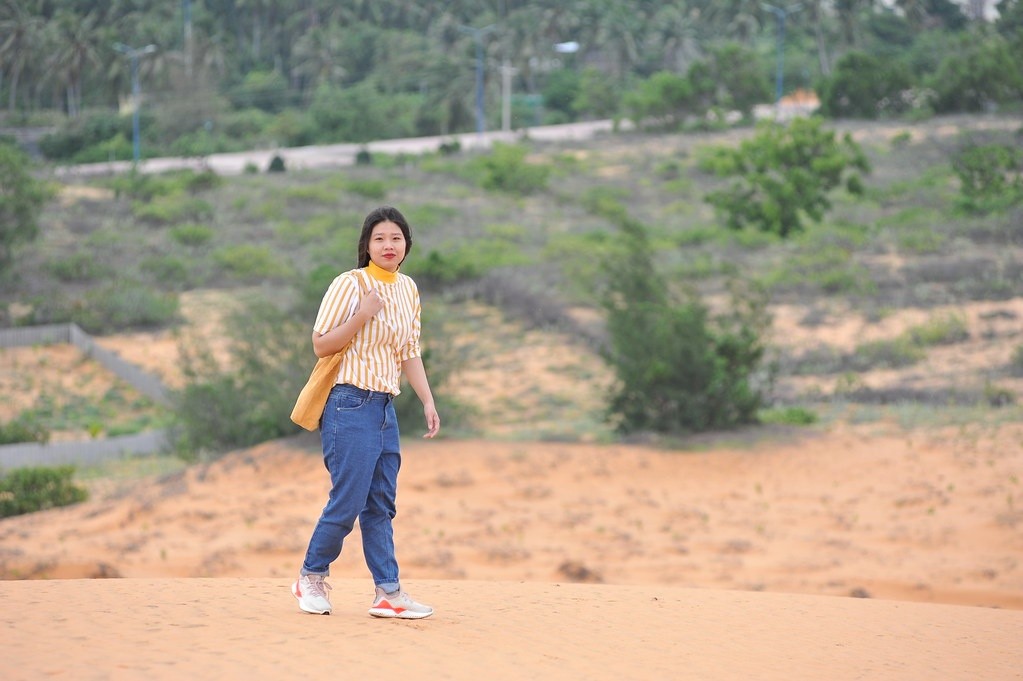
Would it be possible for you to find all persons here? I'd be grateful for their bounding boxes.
[293,207,440,619]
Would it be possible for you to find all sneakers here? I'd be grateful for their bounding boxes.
[368,586,434,619]
[290,574,334,615]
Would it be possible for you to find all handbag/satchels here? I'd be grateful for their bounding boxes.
[290,268,371,432]
